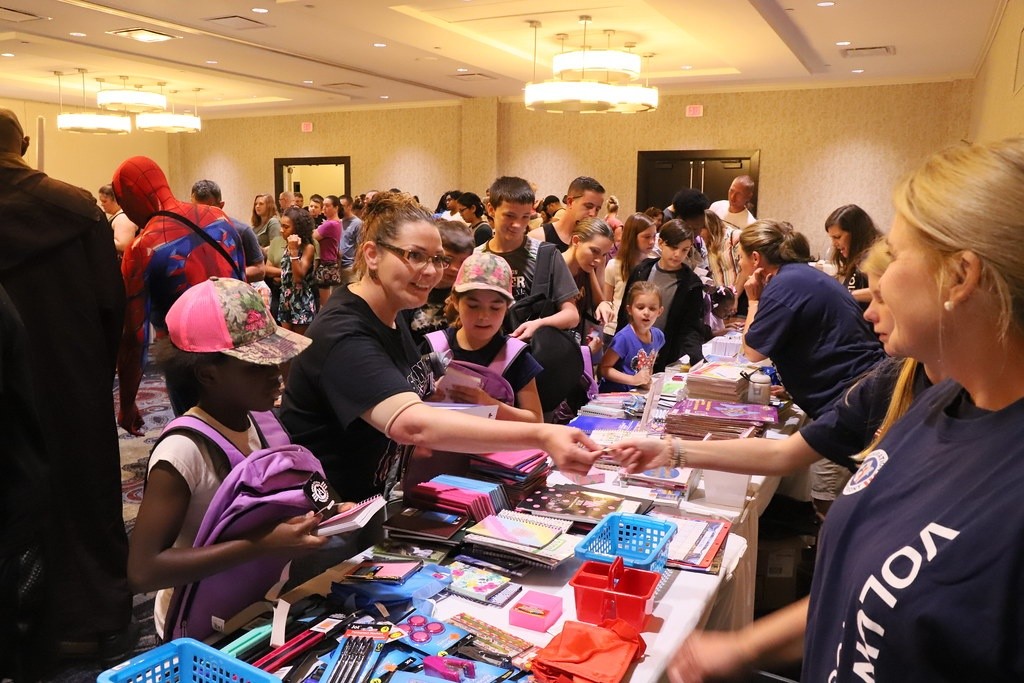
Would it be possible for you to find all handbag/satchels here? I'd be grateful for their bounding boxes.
[583,312,605,366]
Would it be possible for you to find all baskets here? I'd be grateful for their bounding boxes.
[97,638,281,683]
[574,513,678,573]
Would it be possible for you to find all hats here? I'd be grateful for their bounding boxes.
[165,278,313,365]
[453,253,514,300]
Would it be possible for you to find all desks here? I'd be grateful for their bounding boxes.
[205,406,807,683]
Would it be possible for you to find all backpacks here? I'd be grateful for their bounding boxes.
[398,332,527,488]
[553,345,599,424]
[509,242,585,411]
[142,409,327,642]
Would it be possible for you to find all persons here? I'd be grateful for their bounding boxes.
[113,157,247,438]
[276,190,605,545]
[0,110,127,683]
[99,175,760,440]
[599,176,952,561]
[128,279,361,645]
[676,437,686,469]
[666,138,1024,683]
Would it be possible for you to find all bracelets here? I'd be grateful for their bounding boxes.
[668,438,679,470]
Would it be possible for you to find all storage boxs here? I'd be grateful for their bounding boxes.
[574,510,679,573]
[509,590,563,634]
[96,637,283,683]
[702,465,752,507]
[569,560,661,632]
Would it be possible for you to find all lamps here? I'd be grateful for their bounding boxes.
[524,14,660,117]
[53,68,203,136]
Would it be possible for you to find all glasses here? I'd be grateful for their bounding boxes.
[458,207,468,213]
[376,241,451,270]
[445,198,453,202]
[562,195,583,204]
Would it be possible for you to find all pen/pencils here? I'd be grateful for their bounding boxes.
[325,637,375,683]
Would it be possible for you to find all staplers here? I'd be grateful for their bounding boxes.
[423,656,474,683]
[458,642,512,668]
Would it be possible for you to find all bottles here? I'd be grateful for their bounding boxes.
[747,368,771,406]
[421,348,453,379]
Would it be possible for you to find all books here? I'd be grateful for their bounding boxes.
[341,416,643,608]
